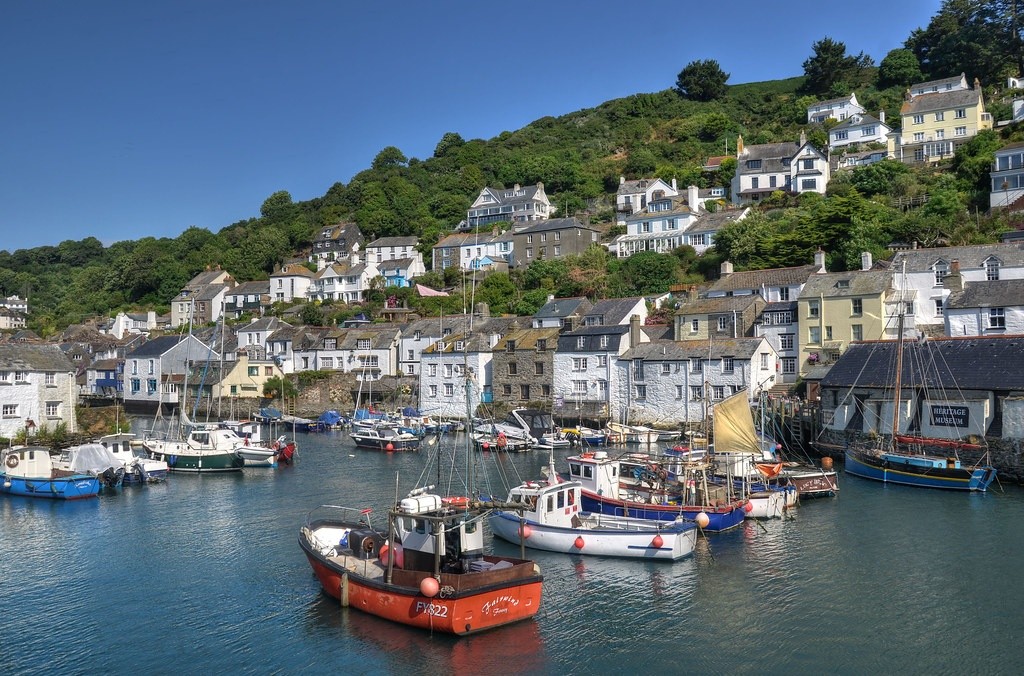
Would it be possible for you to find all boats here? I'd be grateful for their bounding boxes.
[0,213,839,639]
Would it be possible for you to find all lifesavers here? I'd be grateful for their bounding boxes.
[629,454,649,462]
[441,497,469,509]
[580,453,594,459]
[673,444,690,452]
[497,436,506,446]
[527,480,550,487]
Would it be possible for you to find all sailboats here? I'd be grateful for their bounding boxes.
[807,260,997,493]
